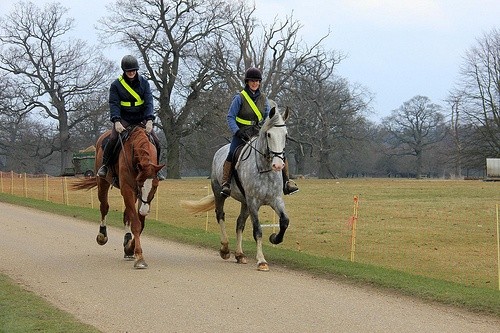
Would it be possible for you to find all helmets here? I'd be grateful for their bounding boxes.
[121,55,139,72]
[244,67,262,83]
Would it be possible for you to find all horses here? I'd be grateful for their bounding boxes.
[181,105,290,272]
[68,125,166,269]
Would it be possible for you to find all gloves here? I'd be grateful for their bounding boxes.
[115,122,126,132]
[236,130,247,139]
[145,120,153,133]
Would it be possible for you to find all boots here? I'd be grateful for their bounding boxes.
[98,142,114,178]
[157,171,165,181]
[222,160,232,197]
[282,158,299,195]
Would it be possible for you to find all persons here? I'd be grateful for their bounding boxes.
[220,67,299,199]
[96,54,166,181]
